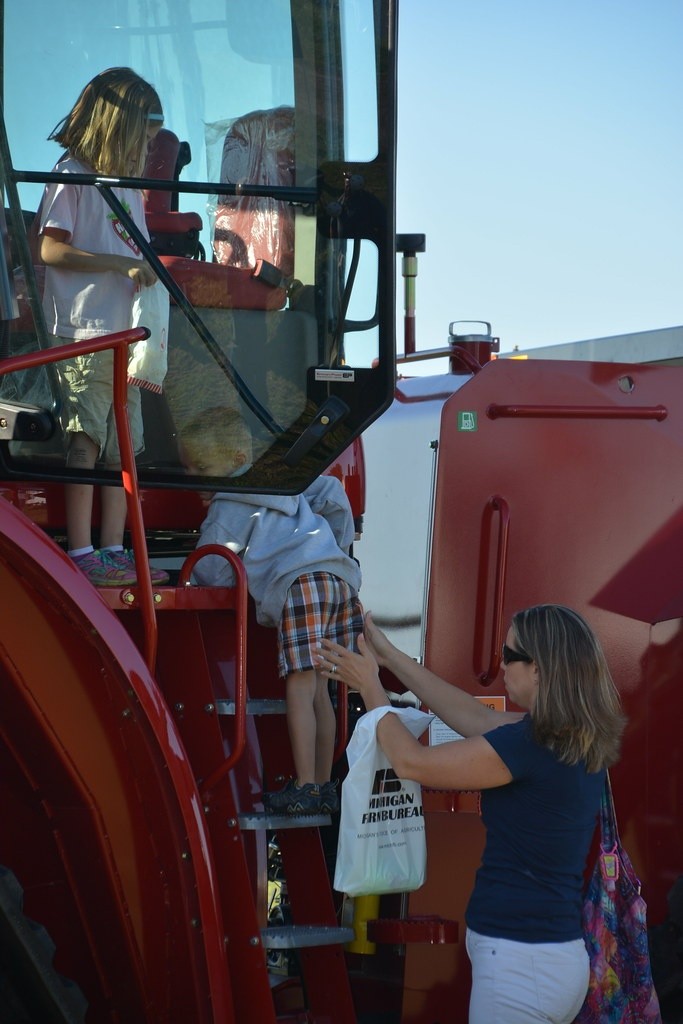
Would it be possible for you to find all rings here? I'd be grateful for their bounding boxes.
[332,664,337,672]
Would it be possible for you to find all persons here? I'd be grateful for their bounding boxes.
[34,65,170,585]
[312,603,630,1024]
[179,406,363,813]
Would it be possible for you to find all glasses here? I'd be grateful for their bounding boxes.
[502,645,536,665]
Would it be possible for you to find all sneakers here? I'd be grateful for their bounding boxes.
[103,550,170,585]
[261,779,321,814]
[72,549,138,586]
[319,778,339,814]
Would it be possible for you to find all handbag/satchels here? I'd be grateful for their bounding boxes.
[127,275,169,395]
[579,762,662,1024]
[334,707,437,898]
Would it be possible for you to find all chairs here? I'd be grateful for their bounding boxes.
[11,129,203,331]
[158,106,296,312]
